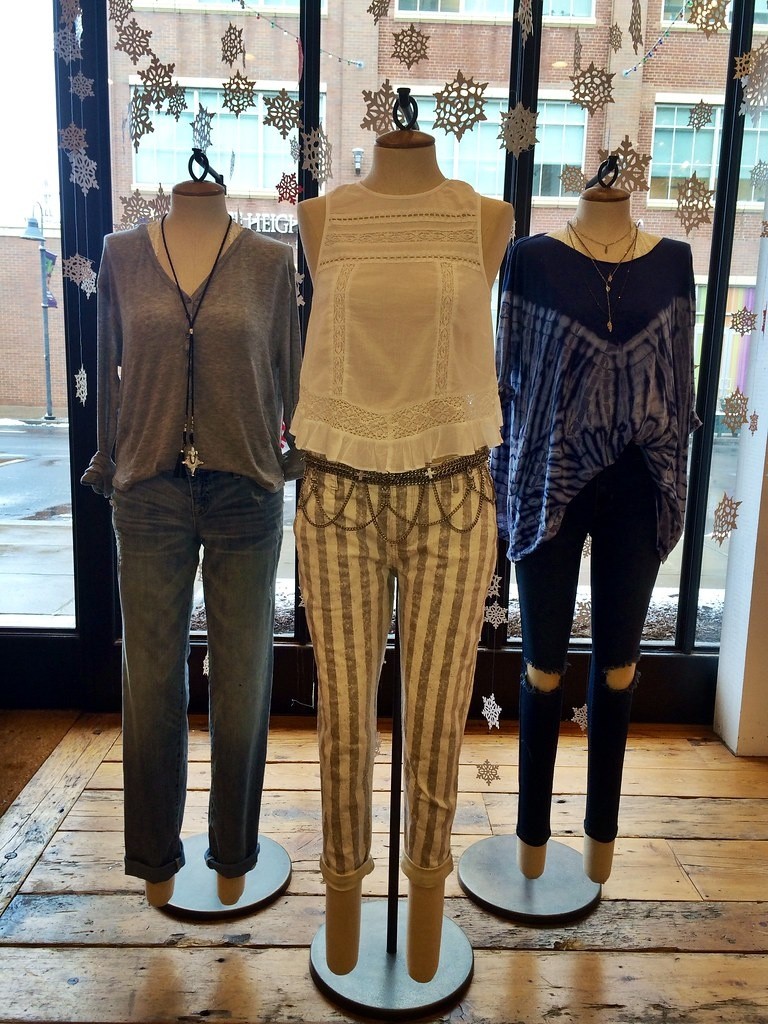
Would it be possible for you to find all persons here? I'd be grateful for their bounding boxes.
[81,180,302,906]
[295,128,515,983]
[491,186,702,883]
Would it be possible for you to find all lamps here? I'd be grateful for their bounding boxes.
[352,148,364,175]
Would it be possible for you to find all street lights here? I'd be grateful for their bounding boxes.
[21,200,58,421]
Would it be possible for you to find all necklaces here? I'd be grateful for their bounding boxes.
[161,212,233,479]
[566,221,639,333]
